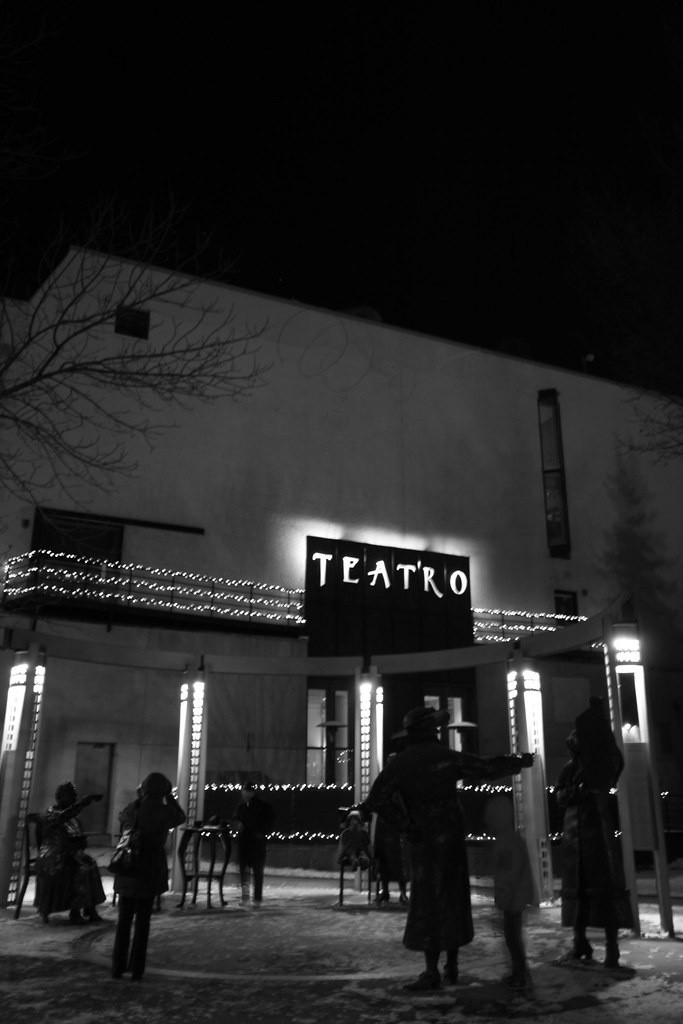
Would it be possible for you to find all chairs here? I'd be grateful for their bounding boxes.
[14,812,66,921]
[336,808,380,905]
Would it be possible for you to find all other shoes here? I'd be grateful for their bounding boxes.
[443,964,458,985]
[399,893,408,904]
[503,972,526,986]
[112,968,122,979]
[131,969,143,981]
[352,858,359,871]
[241,884,250,901]
[84,906,103,922]
[360,857,369,870]
[374,892,389,902]
[69,914,88,924]
[411,969,441,990]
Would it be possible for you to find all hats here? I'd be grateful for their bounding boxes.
[402,707,449,734]
[347,810,362,821]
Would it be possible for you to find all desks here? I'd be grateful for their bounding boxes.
[178,825,233,908]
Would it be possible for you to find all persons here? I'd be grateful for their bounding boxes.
[474,794,540,989]
[112,772,185,983]
[231,780,275,908]
[367,707,535,991]
[337,810,410,908]
[550,697,635,971]
[34,782,106,925]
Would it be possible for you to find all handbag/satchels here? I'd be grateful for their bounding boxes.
[111,829,157,879]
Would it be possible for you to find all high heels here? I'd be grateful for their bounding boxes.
[567,939,594,961]
[605,944,619,967]
